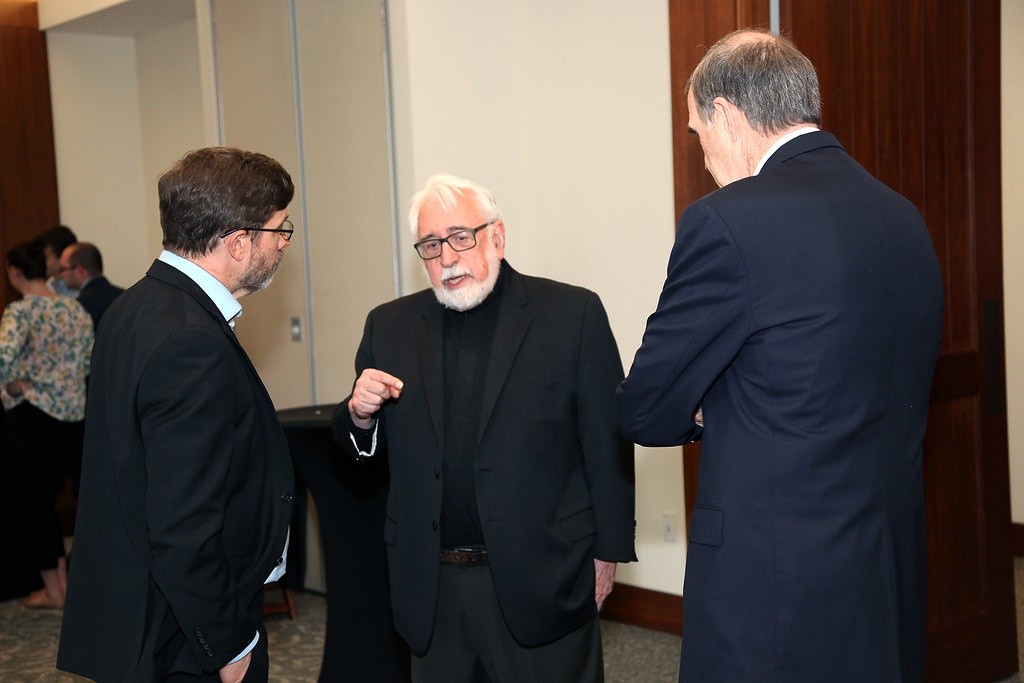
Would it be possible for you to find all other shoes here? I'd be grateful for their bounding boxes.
[23,590,65,610]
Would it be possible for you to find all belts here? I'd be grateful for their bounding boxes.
[440,547,488,568]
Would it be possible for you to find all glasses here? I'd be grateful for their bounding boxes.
[57,264,77,274]
[413,222,496,260]
[220,219,294,240]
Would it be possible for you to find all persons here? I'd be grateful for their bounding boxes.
[56,144,295,683]
[0,225,126,609]
[331,174,639,683]
[614,29,947,683]
